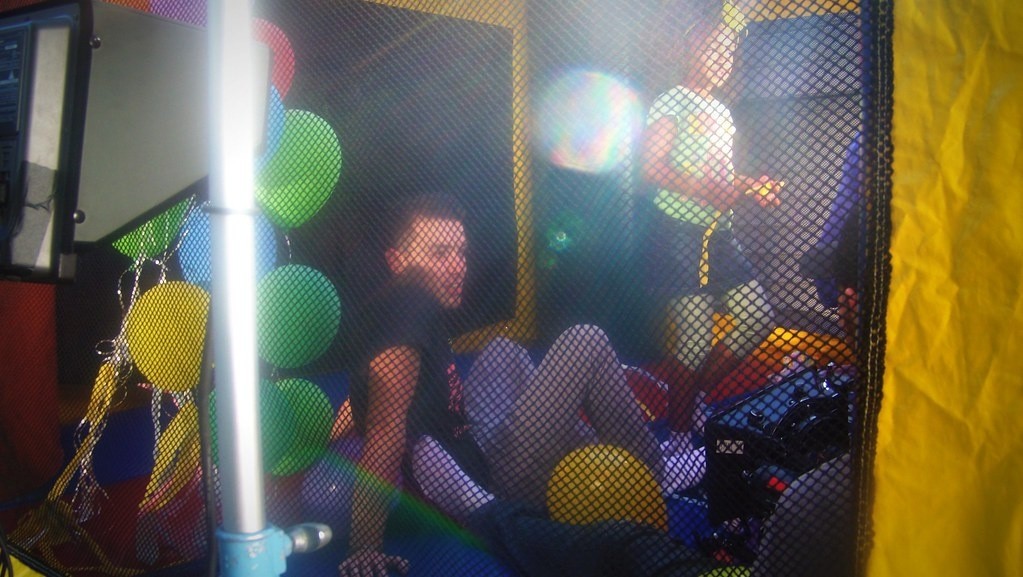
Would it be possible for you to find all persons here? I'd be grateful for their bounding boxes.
[638,18,789,436]
[328,192,759,577]
